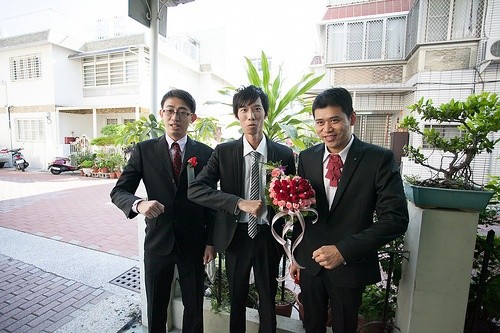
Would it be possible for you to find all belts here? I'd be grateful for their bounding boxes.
[238,223,272,233]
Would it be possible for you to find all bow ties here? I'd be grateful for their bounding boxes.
[325,154,343,187]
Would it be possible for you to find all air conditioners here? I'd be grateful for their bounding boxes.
[485,37,500,60]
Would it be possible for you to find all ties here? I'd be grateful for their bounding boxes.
[172,143,182,180]
[248,151,260,239]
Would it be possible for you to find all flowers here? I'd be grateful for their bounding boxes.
[261,160,288,178]
[188,157,198,168]
[265,174,317,223]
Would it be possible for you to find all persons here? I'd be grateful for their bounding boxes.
[187,85,296,333]
[290,88,409,333]
[110,89,217,333]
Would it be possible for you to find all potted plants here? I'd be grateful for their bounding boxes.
[275,287,297,318]
[357,284,398,333]
[80,160,122,179]
[397,91,500,212]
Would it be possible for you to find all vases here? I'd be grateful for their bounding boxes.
[296,292,332,326]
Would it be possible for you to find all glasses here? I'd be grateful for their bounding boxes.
[163,109,193,117]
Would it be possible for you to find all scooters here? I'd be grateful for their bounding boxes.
[47,155,84,175]
[8,148,29,172]
